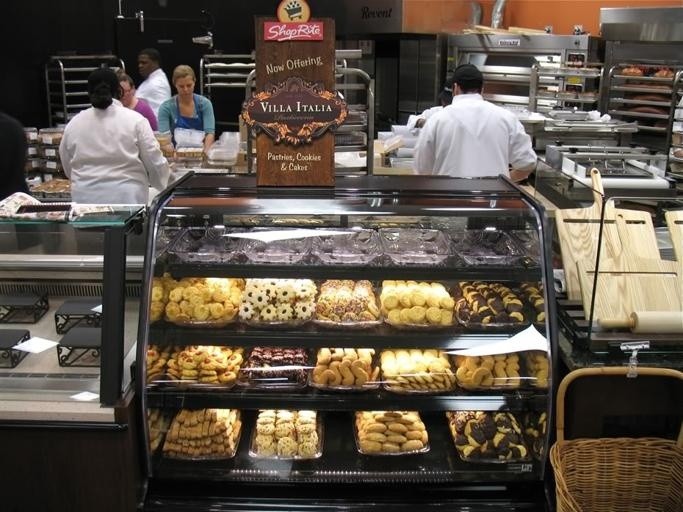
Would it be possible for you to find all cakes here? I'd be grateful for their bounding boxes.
[244,346,308,389]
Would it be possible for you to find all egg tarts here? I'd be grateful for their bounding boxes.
[166,286,240,323]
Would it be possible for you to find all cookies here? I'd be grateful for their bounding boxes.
[455,282,524,325]
[519,282,547,322]
[238,280,315,323]
[316,279,381,324]
[526,346,549,391]
[166,345,243,386]
[256,410,319,459]
[380,278,454,326]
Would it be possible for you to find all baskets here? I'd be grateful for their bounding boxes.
[546,362,683,511]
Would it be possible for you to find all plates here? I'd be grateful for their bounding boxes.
[452,360,522,393]
[445,412,532,466]
[236,348,306,392]
[161,427,242,462]
[162,370,236,393]
[239,314,313,329]
[309,347,380,393]
[314,311,384,331]
[381,374,458,396]
[350,412,431,459]
[170,224,243,265]
[165,311,236,328]
[316,225,384,264]
[378,280,456,329]
[380,225,451,265]
[245,226,314,264]
[454,308,515,333]
[247,410,324,462]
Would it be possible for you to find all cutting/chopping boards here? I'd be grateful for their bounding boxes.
[555,162,661,303]
[573,212,683,321]
[666,210,683,275]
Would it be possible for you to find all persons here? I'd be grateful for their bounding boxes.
[0,110,46,251]
[130,49,174,125]
[58,68,175,256]
[114,74,158,131]
[406,81,452,132]
[159,66,215,154]
[414,64,538,186]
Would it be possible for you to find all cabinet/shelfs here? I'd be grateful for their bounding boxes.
[0,200,148,512]
[135,172,558,512]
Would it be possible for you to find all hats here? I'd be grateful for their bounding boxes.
[447,60,485,90]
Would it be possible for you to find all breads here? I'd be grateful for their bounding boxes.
[162,411,236,460]
[380,351,454,392]
[653,67,676,82]
[317,348,377,388]
[356,409,427,454]
[447,406,528,461]
[456,353,520,388]
[621,67,641,76]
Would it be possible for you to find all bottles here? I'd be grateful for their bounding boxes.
[526,410,547,463]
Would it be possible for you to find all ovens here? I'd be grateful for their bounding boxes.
[601,7,683,154]
[443,30,597,110]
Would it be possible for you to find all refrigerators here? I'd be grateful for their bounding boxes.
[399,34,446,126]
